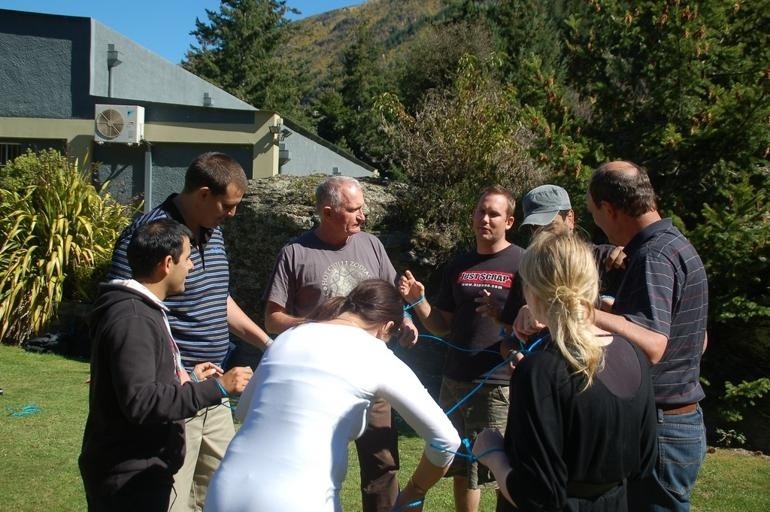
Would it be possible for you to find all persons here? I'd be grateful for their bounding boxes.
[108,152,275,512]
[473,229,659,511]
[503,185,631,341]
[78,217,253,510]
[205,278,461,512]
[400,182,525,510]
[514,161,710,510]
[267,177,401,512]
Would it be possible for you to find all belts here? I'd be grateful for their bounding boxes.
[660,403,702,418]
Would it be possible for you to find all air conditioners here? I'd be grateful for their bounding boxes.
[93,104,144,146]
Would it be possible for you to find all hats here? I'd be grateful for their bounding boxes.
[519,185,573,232]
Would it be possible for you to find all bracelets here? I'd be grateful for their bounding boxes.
[410,474,427,497]
